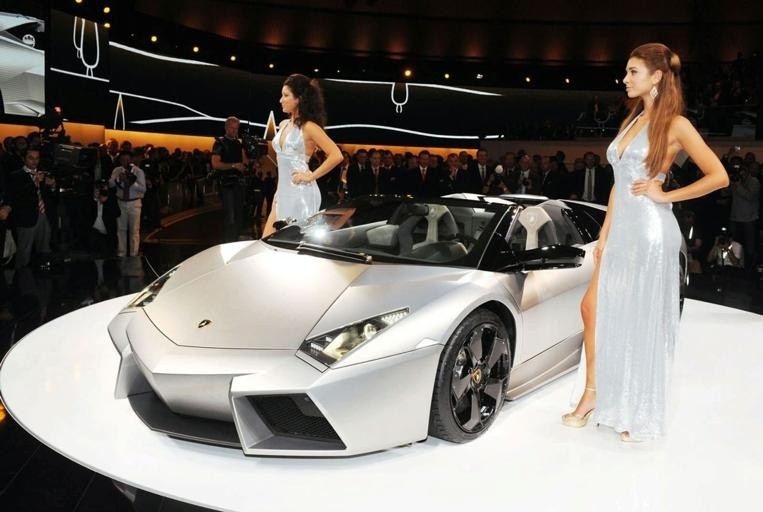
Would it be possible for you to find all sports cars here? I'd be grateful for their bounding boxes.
[0,12,47,125]
[107,188,688,459]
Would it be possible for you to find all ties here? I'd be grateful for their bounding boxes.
[360,165,364,172]
[422,168,425,184]
[519,171,524,194]
[340,168,346,194]
[587,170,592,202]
[123,170,130,201]
[482,165,485,188]
[31,171,45,215]
[374,168,377,193]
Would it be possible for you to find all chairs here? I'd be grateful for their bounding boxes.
[502,204,558,254]
[405,202,459,249]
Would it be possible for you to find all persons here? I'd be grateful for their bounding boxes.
[659,149,762,315]
[307,143,618,212]
[562,42,731,444]
[2,116,279,291]
[262,72,343,242]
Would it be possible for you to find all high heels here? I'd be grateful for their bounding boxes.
[562,386,602,431]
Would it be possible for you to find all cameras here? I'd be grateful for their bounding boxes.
[100,189,108,196]
[733,144,741,152]
[523,178,529,186]
[719,227,729,245]
[39,172,48,177]
[125,163,137,185]
[731,164,740,182]
